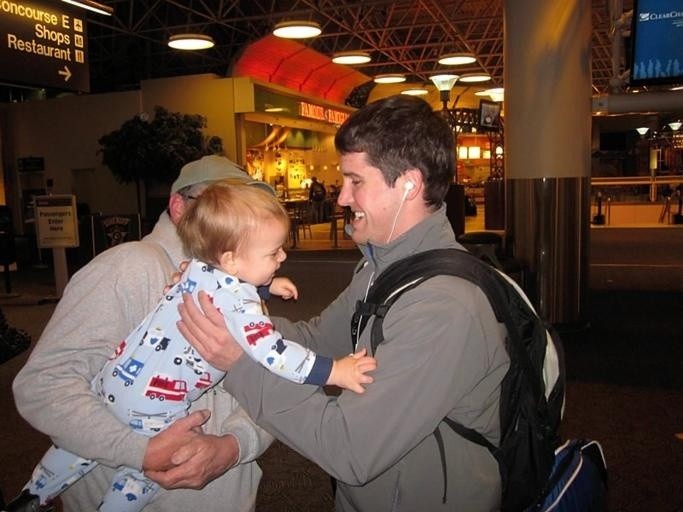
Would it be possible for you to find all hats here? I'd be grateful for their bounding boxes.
[170,155,276,196]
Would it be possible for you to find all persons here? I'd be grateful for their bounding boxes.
[308,176,326,225]
[164,94,511,512]
[11,155,262,512]
[11,182,378,509]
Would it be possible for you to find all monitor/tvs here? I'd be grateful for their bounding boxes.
[629,0,683,89]
[479,99,500,132]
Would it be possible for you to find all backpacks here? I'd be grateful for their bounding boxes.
[350,247,609,512]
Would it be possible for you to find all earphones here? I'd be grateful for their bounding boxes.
[403,181,413,201]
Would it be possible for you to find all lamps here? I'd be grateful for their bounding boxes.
[459,72,491,82]
[429,75,461,120]
[635,127,649,137]
[373,74,406,84]
[668,122,683,132]
[273,19,321,40]
[330,50,371,65]
[438,53,477,66]
[168,34,216,51]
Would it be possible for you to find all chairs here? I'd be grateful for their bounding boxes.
[329,198,352,248]
[284,198,313,248]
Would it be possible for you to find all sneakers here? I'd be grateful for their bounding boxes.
[0,488,62,512]
